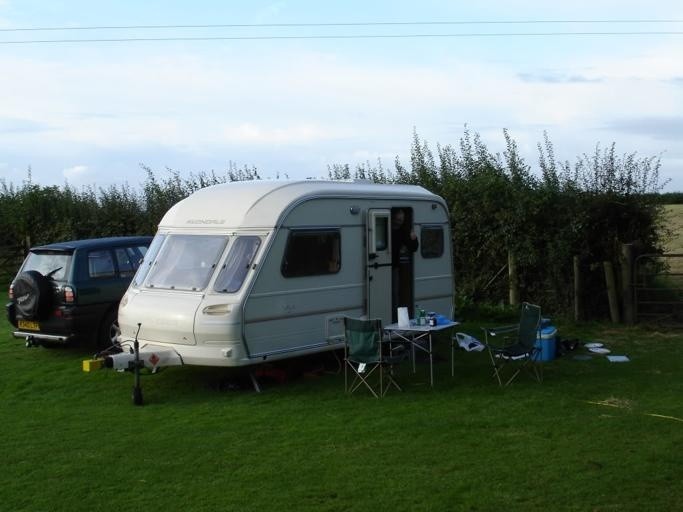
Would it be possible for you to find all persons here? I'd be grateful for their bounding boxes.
[299,239,342,274]
[393,209,418,320]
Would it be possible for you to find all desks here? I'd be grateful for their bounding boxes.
[383,318,460,388]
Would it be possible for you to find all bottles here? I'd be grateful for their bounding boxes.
[415,305,426,326]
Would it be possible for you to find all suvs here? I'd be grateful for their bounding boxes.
[5,235,154,351]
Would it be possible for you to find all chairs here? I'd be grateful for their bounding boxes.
[479,302,544,391]
[343,316,405,400]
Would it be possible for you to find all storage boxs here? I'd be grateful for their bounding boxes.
[532,326,558,361]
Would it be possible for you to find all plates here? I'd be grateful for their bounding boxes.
[585,343,611,354]
